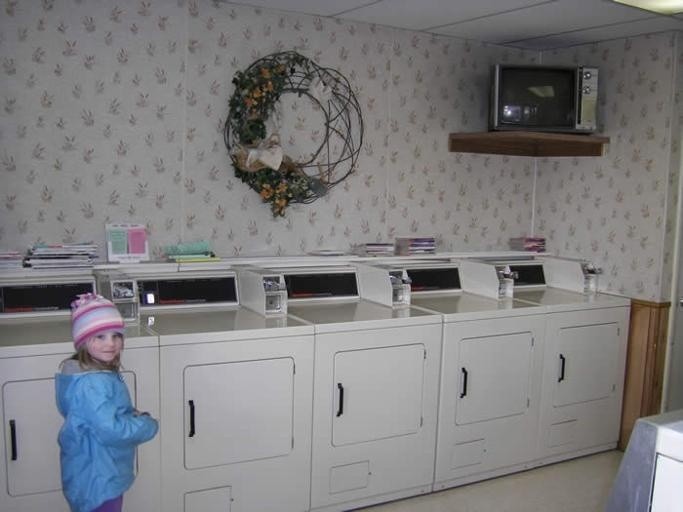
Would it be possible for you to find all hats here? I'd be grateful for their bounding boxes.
[70,291,125,351]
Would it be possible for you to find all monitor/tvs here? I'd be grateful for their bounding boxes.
[488,62,599,135]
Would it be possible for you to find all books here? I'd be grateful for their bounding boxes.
[309,234,437,259]
[0,228,223,273]
[509,236,549,252]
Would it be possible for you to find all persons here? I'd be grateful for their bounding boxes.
[54,292,158,512]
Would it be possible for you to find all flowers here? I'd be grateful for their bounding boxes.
[228,44,313,217]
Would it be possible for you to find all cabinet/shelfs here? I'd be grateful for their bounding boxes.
[538,297,635,465]
[156,305,311,511]
[310,297,433,511]
[1,319,161,512]
[433,305,539,491]
